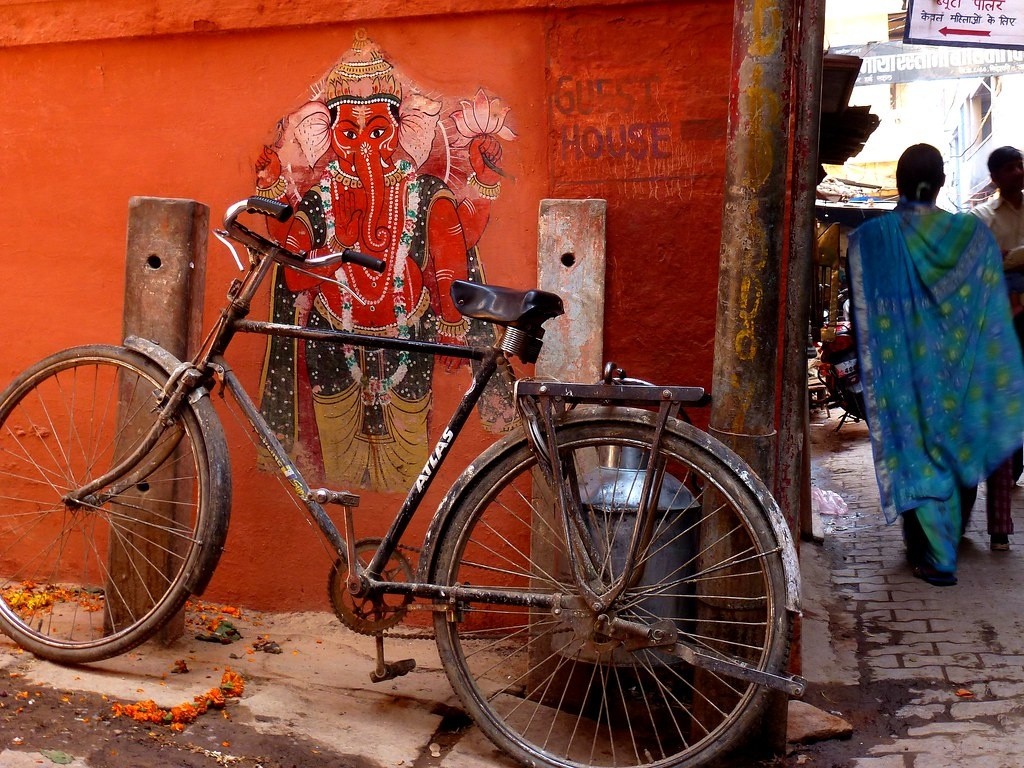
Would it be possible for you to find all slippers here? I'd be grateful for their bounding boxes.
[913,562,958,585]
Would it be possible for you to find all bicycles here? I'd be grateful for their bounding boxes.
[0,196,807,768]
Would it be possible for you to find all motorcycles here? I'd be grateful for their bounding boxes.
[817,309,870,434]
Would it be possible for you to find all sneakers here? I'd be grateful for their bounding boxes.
[991,534,1009,551]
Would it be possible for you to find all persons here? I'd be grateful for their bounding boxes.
[845,143,1024,585]
[965,146,1024,552]
